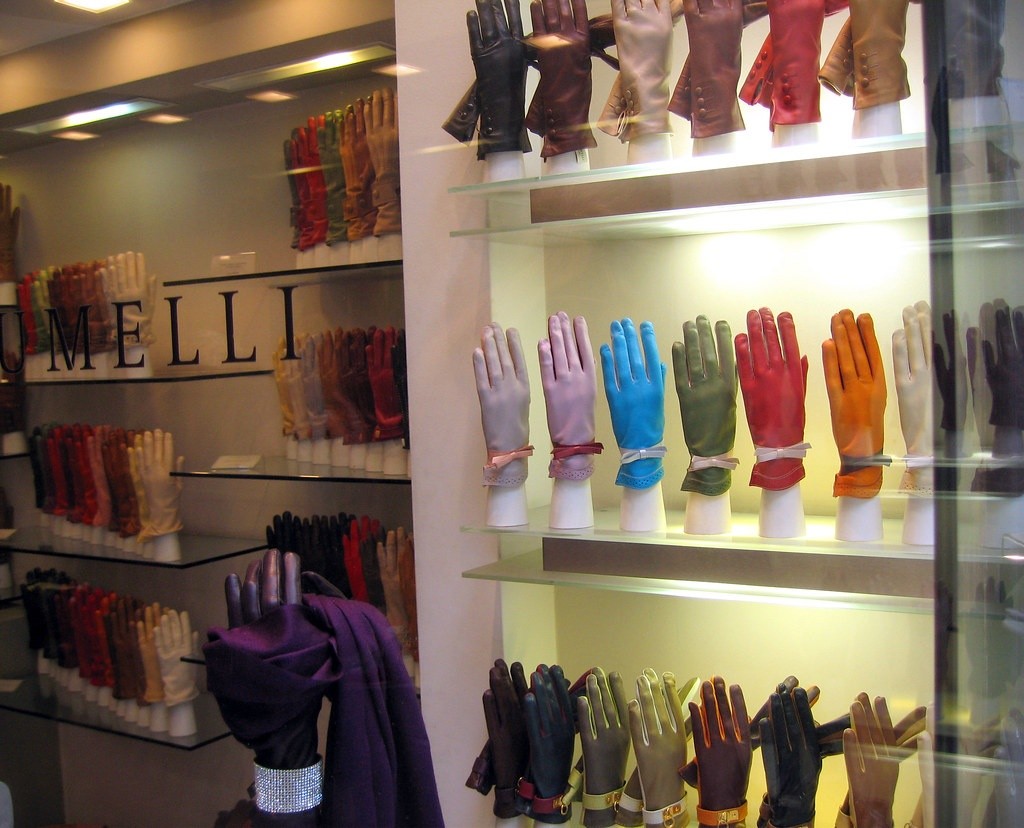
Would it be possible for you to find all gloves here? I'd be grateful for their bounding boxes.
[0,350,26,434]
[266,510,420,664]
[17,252,158,357]
[19,567,200,707]
[225,548,348,748]
[272,325,410,450]
[441,0,1005,158]
[466,659,1024,828]
[27,423,185,542]
[472,299,1024,496]
[283,85,400,250]
[0,183,20,284]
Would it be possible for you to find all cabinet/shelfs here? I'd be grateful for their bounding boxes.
[392,0,1021,827]
[0,0,418,828]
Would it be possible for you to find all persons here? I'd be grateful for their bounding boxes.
[16,0,1024,824]
